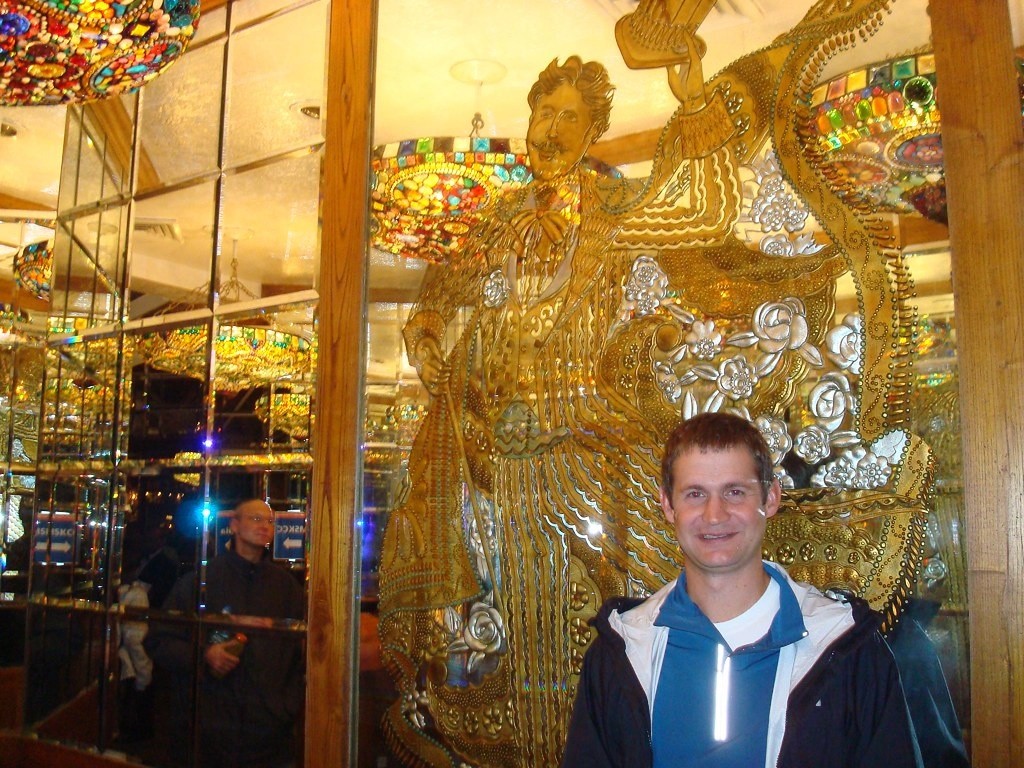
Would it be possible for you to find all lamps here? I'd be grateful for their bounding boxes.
[135,239,316,394]
[368,113,625,262]
[253,395,316,442]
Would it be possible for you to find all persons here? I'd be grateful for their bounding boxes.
[565,412,923,768]
[116,499,309,768]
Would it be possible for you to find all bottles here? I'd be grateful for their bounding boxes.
[210,632,248,678]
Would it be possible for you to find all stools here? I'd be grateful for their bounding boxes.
[0,0,201,107]
[13,237,54,302]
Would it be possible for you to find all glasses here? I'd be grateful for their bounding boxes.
[237,516,274,526]
